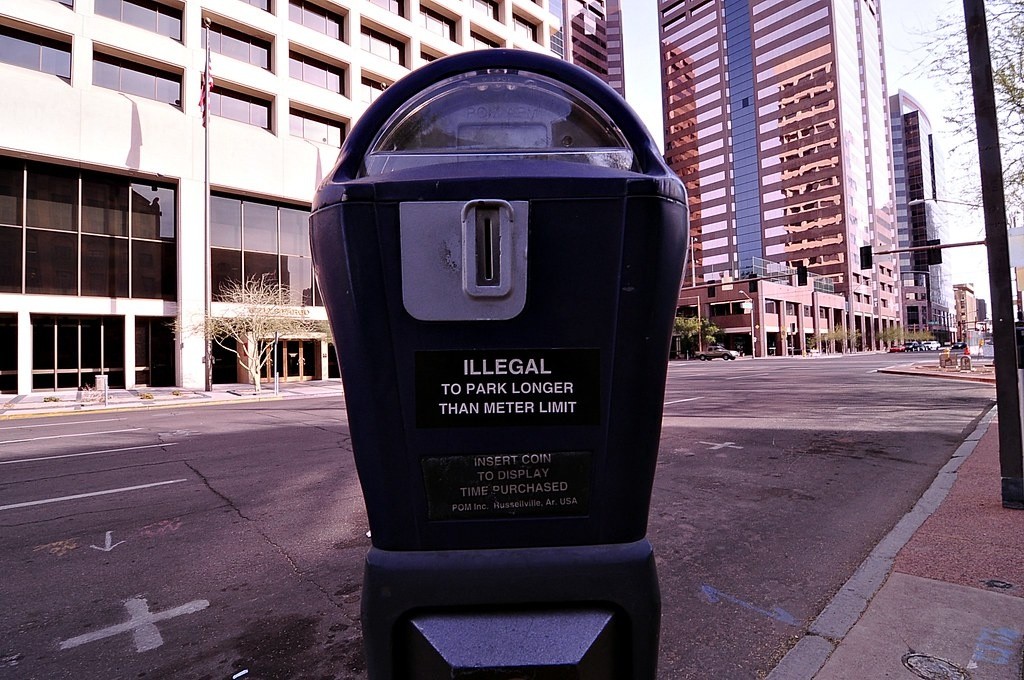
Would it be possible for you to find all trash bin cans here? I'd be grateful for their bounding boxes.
[307,49,689,679]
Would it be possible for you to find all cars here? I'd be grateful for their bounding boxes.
[889,340,941,353]
[951,342,965,349]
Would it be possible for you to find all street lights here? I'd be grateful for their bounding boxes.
[738,289,756,359]
[851,277,871,354]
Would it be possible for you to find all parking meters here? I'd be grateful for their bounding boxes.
[306,39,692,678]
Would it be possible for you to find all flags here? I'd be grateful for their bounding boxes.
[197,44,214,127]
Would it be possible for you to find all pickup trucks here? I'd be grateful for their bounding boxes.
[693,344,740,361]
